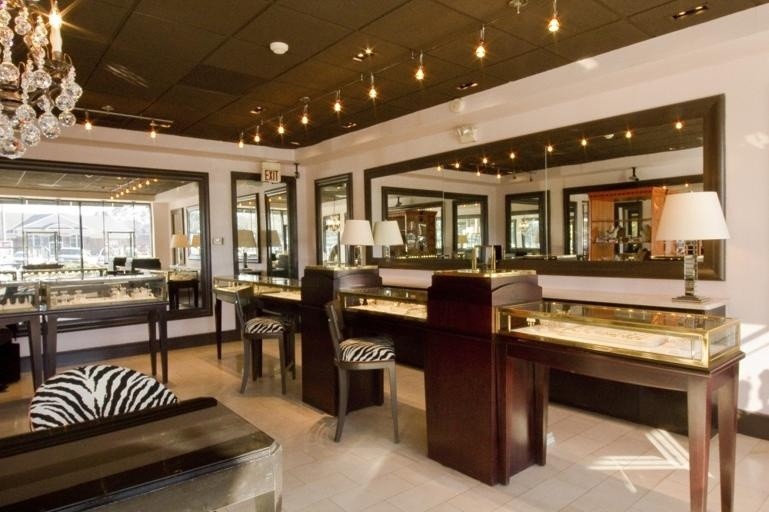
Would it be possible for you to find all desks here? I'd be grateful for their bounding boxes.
[0,395,285,512]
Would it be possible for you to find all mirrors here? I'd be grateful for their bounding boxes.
[313,172,354,266]
[237,193,264,275]
[170,208,186,267]
[1,157,213,338]
[185,204,201,260]
[264,186,290,276]
[361,92,726,280]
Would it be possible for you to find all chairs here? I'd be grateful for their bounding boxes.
[323,299,400,445]
[233,284,295,394]
[25,363,185,433]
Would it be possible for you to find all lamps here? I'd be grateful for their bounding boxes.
[109,178,158,201]
[238,229,258,273]
[655,190,733,305]
[270,229,282,248]
[372,221,404,266]
[437,121,682,184]
[0,0,82,160]
[372,220,403,258]
[341,219,374,266]
[72,107,173,139]
[169,233,188,270]
[190,234,200,246]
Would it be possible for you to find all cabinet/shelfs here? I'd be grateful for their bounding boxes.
[22,258,201,311]
[213,270,747,512]
[0,273,200,394]
